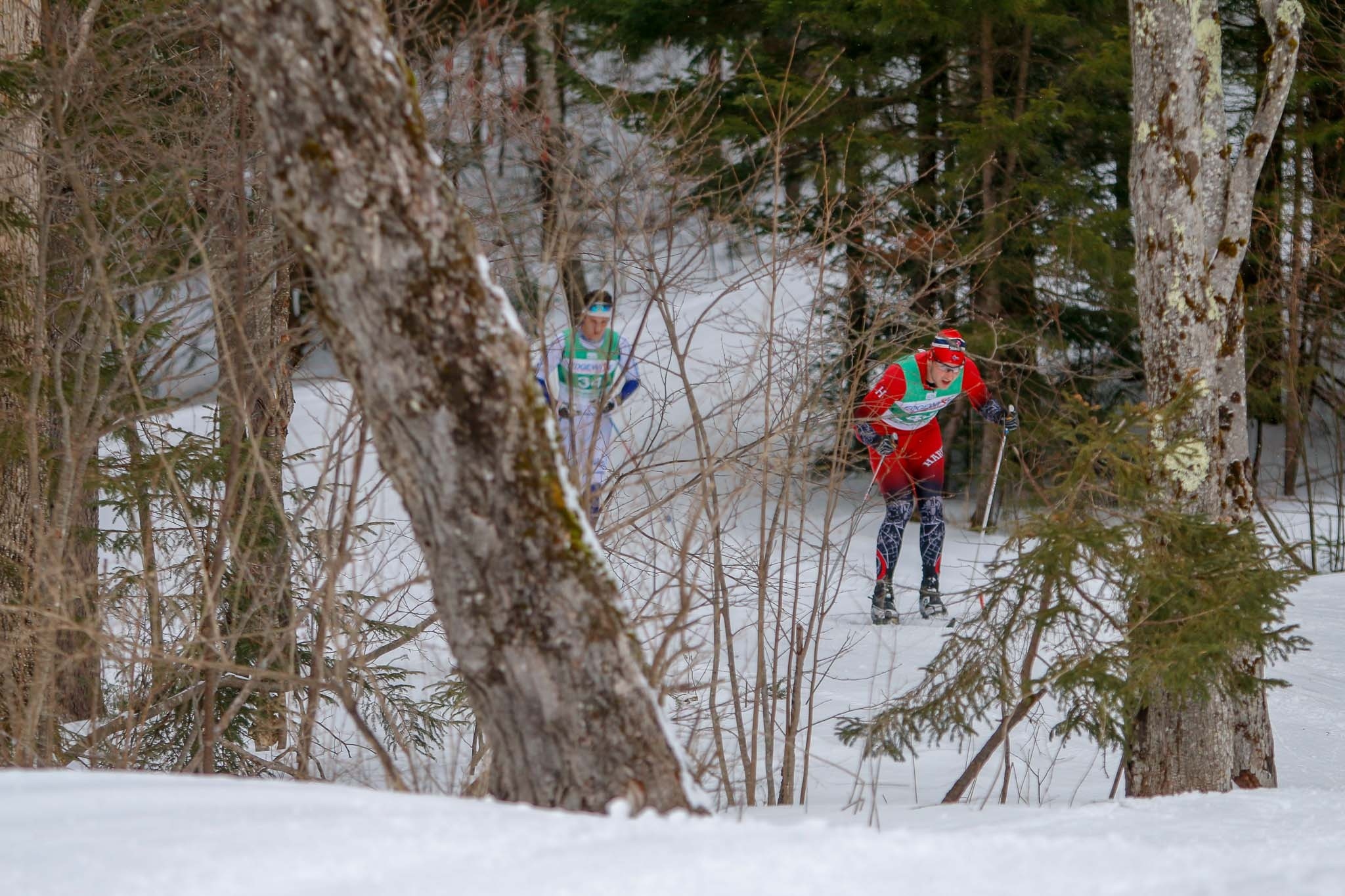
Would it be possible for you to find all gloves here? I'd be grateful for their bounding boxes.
[993,409,1018,431]
[871,433,898,455]
[559,405,571,418]
[603,398,617,414]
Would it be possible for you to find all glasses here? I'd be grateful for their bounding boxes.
[588,304,612,312]
[931,349,965,373]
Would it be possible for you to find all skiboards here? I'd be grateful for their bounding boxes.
[795,612,987,627]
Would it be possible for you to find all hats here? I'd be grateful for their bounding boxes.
[932,329,966,366]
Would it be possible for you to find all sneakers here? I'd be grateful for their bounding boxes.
[870,583,899,625]
[919,587,948,619]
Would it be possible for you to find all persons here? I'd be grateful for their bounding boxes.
[520,87,553,202]
[536,289,640,528]
[848,328,1021,624]
